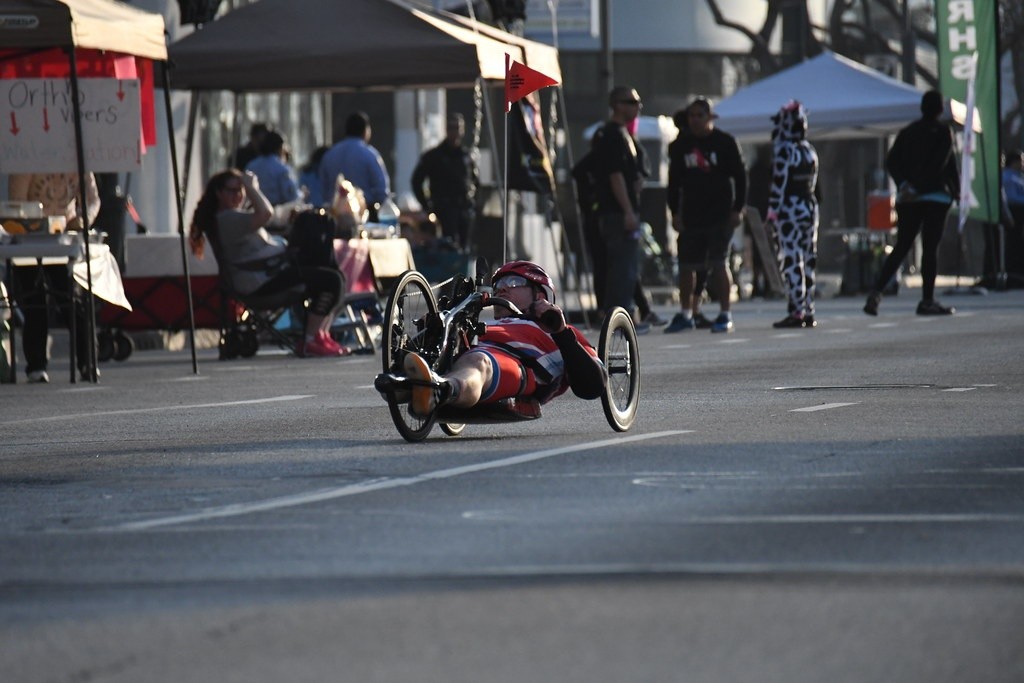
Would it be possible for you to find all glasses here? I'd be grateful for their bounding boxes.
[492,275,529,292]
[622,98,641,106]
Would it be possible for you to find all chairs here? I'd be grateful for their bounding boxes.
[199,216,343,355]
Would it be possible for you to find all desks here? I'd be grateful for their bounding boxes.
[101,232,417,353]
[0,233,108,380]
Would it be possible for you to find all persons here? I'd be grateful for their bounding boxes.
[378,259,608,417]
[9,171,101,382]
[188,85,749,360]
[862,91,962,316]
[1001,152,1024,288]
[764,101,819,328]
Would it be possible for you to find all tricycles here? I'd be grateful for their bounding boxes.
[372,255,641,442]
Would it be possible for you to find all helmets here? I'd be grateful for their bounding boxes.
[491,260,556,295]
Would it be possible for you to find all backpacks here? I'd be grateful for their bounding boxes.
[284,205,337,273]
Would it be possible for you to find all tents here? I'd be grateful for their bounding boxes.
[710,50,992,298]
[150,0,599,325]
[0,0,202,386]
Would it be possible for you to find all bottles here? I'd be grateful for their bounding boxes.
[379,193,401,236]
[634,225,661,257]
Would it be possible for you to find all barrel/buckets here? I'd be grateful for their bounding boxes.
[868,191,895,230]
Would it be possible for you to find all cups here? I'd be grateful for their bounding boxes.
[48,215,65,234]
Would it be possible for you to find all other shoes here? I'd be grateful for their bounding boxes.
[323,331,352,354]
[916,299,955,316]
[405,356,451,415]
[640,310,669,326]
[804,316,815,328]
[772,316,805,328]
[693,313,715,328]
[664,312,694,334]
[610,319,652,336]
[27,371,51,382]
[863,293,881,317]
[710,313,735,332]
[303,330,342,357]
